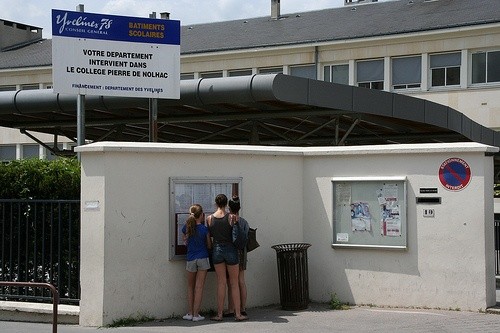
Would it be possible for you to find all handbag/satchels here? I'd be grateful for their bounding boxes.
[247,228,260,252]
[232,214,242,249]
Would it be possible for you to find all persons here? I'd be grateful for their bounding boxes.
[206,193,247,320]
[221,195,252,317]
[182,203,212,321]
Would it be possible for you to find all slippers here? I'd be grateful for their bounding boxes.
[235,316,248,321]
[210,316,223,321]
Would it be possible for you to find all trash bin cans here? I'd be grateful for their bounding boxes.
[271,243,312,311]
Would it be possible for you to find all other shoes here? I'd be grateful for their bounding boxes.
[192,317,205,321]
[222,313,234,317]
[241,311,246,315]
[182,314,193,320]
